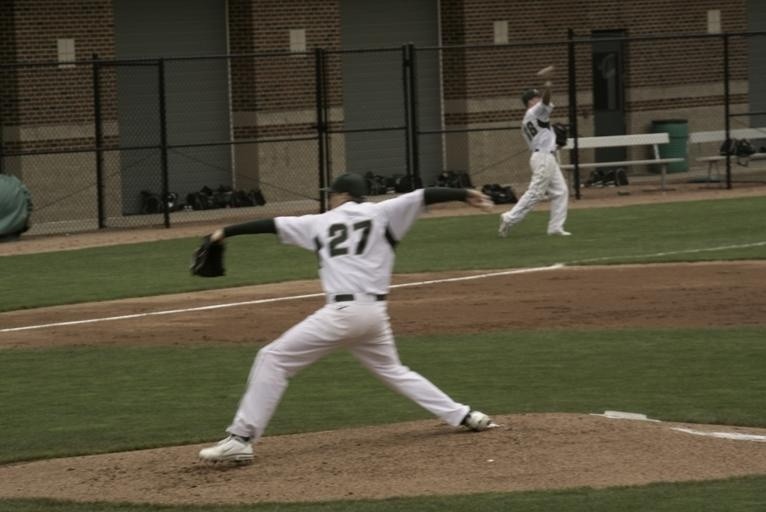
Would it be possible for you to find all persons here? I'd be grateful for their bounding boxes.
[189,170,496,468]
[494,64,575,239]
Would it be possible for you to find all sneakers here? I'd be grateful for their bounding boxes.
[466,411,490,431]
[198,434,254,462]
[498,213,509,238]
[548,230,571,236]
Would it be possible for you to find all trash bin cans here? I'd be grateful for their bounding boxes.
[652,119,689,174]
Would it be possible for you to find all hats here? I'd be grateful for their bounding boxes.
[522,90,540,106]
[319,172,367,197]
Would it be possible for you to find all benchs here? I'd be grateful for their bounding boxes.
[550,126,764,197]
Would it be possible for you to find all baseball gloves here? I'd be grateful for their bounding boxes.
[553,123,568,148]
[191,237,229,279]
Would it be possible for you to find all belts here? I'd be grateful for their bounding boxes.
[332,292,387,300]
[533,146,557,154]
